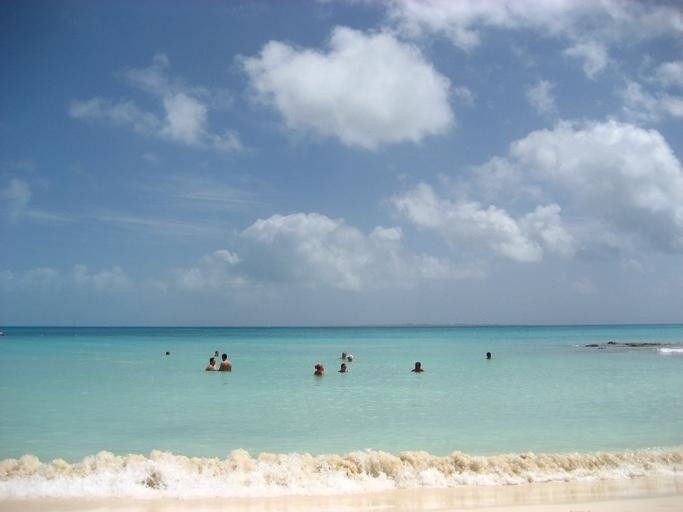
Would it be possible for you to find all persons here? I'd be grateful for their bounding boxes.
[342,352,347,358]
[166,352,170,355]
[338,363,347,372]
[219,354,232,371]
[347,355,353,362]
[315,364,324,375]
[215,351,219,356]
[206,357,218,371]
[411,362,424,371]
[487,352,491,359]
